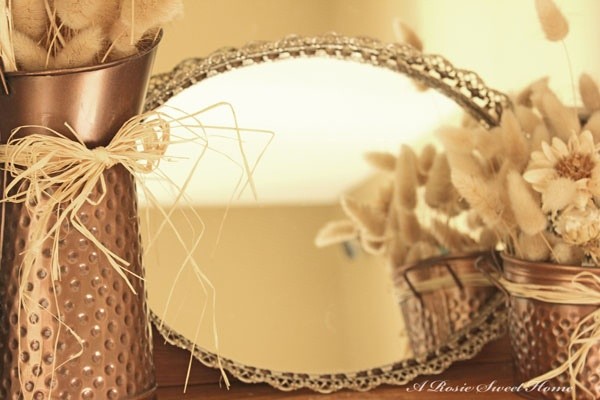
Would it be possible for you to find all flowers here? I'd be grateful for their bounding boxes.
[314,123,502,268]
[435,1,600,264]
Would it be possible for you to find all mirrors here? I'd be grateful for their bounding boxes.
[128,32,512,396]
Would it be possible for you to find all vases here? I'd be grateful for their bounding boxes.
[387,243,497,363]
[497,247,600,400]
[1,38,167,399]
